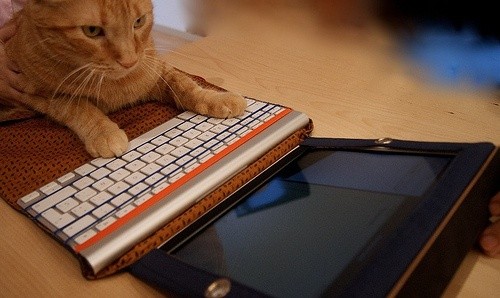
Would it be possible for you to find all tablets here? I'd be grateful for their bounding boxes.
[126,136,496,297]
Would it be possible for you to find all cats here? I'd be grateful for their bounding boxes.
[0,0,247,159]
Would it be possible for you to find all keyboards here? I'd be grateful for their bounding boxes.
[21,92,309,276]
